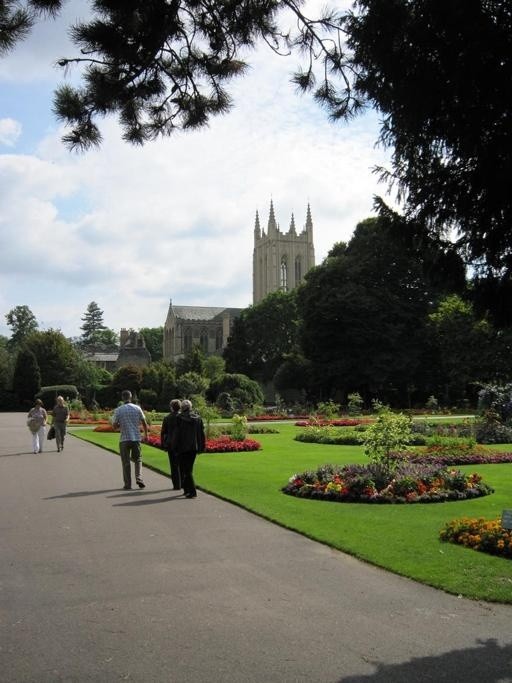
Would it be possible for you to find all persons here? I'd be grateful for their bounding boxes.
[171,400,206,498]
[111,390,149,489]
[161,399,183,490]
[51,395,70,452]
[28,399,48,454]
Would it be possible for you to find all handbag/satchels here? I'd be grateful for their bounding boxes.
[28,419,41,432]
[46,424,57,441]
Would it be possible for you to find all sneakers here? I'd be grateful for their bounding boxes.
[123,480,146,489]
[173,483,197,499]
[33,447,64,454]
